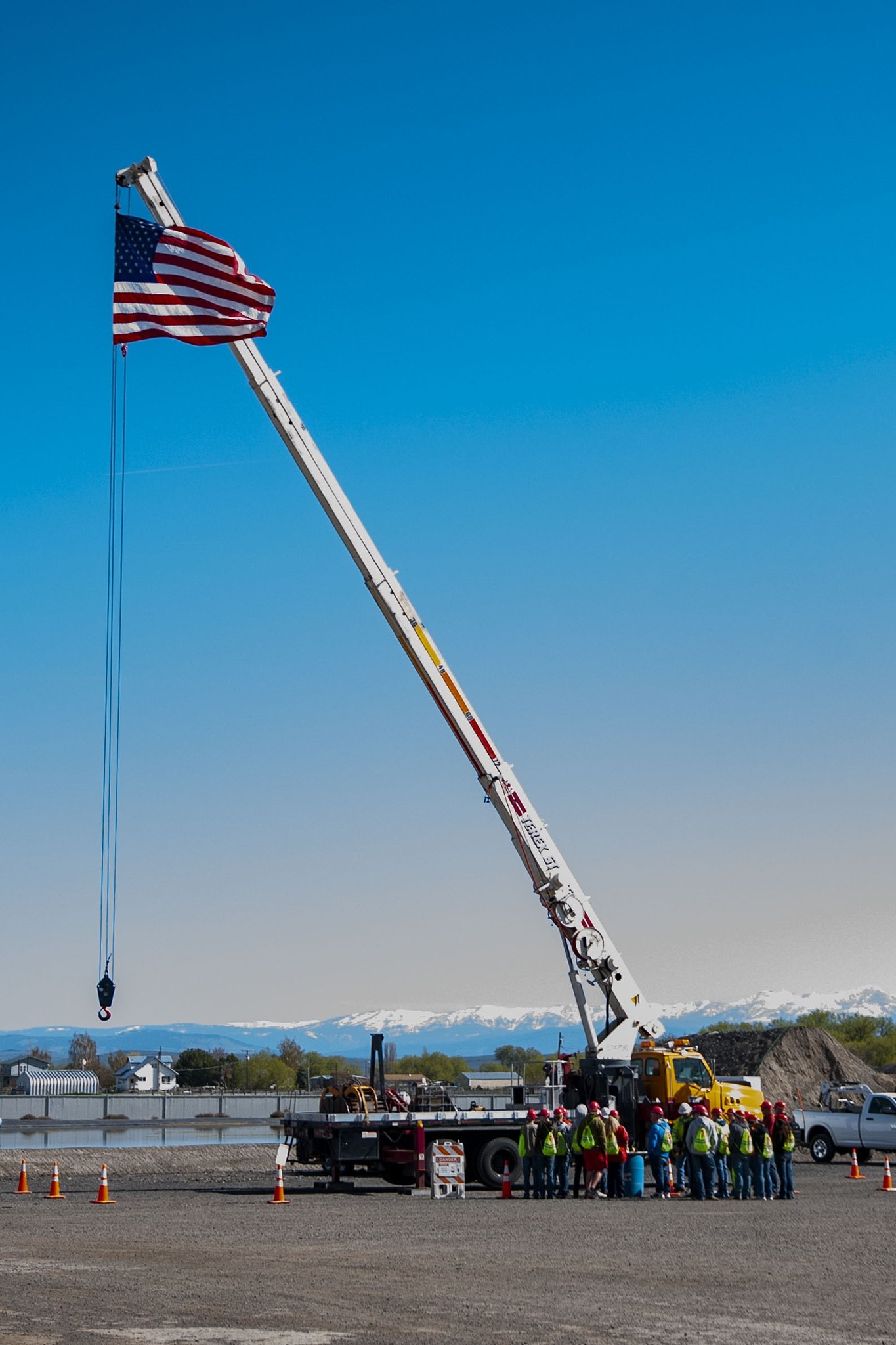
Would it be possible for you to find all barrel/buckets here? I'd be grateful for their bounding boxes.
[624,1154,645,1198]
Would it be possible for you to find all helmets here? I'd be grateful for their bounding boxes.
[526,1101,619,1120]
[651,1107,664,1115]
[678,1101,788,1123]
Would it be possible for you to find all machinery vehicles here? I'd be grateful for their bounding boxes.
[95,154,768,1183]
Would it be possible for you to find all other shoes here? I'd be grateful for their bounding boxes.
[523,1190,607,1199]
[650,1186,795,1201]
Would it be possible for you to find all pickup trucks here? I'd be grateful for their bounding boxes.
[790,1079,896,1165]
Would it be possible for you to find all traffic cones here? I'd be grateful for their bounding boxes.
[496,1158,519,1199]
[668,1157,680,1196]
[13,1158,33,1194]
[44,1161,67,1199]
[266,1164,291,1204]
[88,1164,116,1203]
[844,1147,867,1179]
[879,1156,896,1192]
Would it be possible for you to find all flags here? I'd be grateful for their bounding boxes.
[113,212,276,347]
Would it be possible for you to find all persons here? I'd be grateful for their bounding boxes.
[521,1100,801,1201]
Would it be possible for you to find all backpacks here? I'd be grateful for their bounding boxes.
[732,1123,754,1154]
[556,1132,568,1156]
[580,1117,601,1149]
[755,1128,774,1158]
[781,1129,795,1151]
[692,1121,713,1151]
[518,1125,526,1157]
[539,1122,557,1155]
[606,1130,620,1155]
[655,1123,673,1152]
[714,1123,731,1155]
[572,1119,584,1154]
